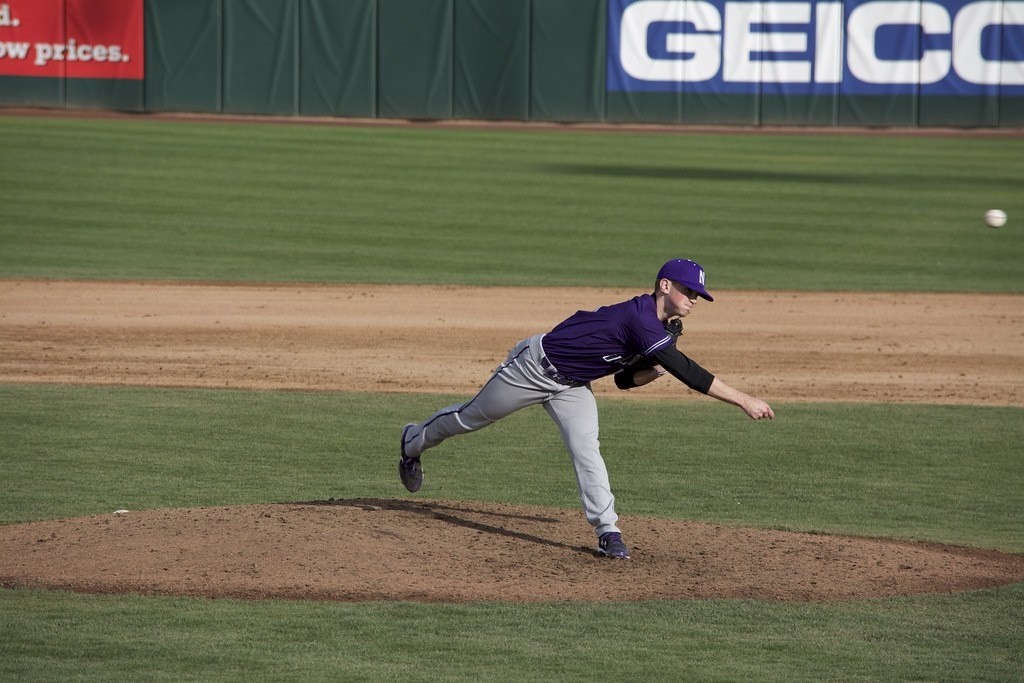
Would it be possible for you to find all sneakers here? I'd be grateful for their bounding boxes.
[398,424,424,492]
[597,532,630,560]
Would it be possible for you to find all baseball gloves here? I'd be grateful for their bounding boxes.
[646,315,685,367]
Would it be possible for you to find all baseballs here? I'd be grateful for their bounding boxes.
[986,208,1007,228]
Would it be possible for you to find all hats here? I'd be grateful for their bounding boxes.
[657,258,714,302]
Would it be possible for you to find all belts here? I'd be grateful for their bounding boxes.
[541,357,574,385]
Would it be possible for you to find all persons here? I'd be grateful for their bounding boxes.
[395,258,775,561]
